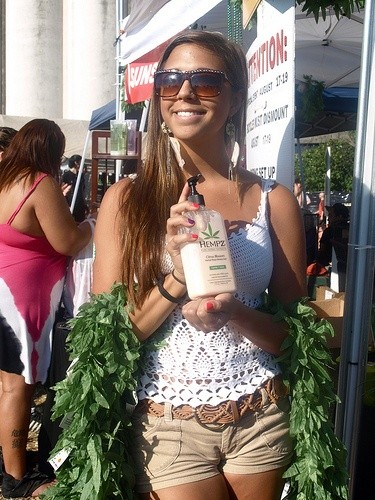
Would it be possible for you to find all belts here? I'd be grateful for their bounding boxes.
[133,373,288,430]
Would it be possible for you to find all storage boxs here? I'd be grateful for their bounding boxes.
[305,285,345,351]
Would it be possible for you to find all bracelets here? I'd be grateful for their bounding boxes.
[78,218,96,234]
[157,268,188,304]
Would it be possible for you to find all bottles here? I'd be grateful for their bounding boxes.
[119,173,138,184]
[107,173,113,187]
[97,172,103,203]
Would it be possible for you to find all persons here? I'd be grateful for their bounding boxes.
[92,30,305,500]
[1,126,91,223]
[1,118,99,497]
[289,173,352,276]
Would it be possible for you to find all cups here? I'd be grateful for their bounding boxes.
[110,120,126,155]
[126,120,137,156]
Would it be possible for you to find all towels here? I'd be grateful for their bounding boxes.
[62,216,99,318]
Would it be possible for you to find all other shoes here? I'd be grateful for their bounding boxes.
[1,469,53,497]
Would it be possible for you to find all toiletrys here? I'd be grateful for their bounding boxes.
[176,174,237,301]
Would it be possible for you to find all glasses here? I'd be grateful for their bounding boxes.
[153,68,235,97]
